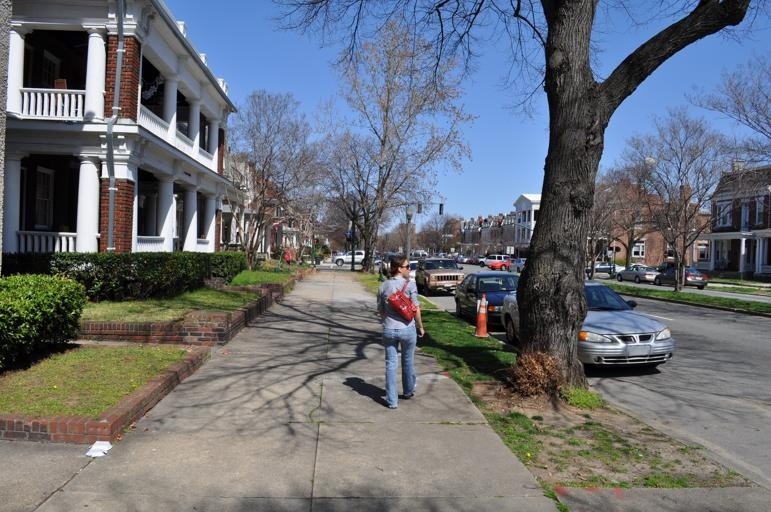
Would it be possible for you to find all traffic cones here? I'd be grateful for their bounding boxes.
[473,292,493,339]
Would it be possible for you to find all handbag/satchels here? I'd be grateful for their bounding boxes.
[388,281,417,321]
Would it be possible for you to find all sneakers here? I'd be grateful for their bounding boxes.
[389,404,398,408]
[403,393,414,399]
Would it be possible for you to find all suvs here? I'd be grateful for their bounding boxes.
[414,255,464,298]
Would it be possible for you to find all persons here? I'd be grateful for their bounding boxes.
[377,255,426,409]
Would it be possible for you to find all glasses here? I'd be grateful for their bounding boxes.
[401,264,410,270]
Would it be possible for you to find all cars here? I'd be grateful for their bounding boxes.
[327,245,612,276]
[454,271,521,328]
[616,263,663,284]
[658,266,709,289]
[500,282,677,369]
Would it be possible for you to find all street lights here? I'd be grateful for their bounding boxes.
[405,205,413,264]
